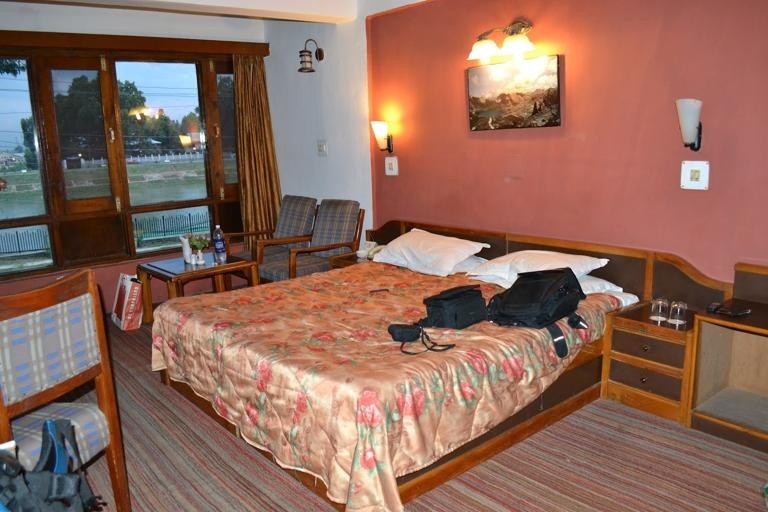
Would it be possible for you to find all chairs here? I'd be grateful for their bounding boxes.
[0,264,135,511]
[221,193,317,294]
[256,197,366,288]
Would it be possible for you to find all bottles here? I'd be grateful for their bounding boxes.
[178,235,197,266]
[212,225,228,263]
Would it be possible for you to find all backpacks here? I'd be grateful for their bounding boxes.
[0,418,107,511]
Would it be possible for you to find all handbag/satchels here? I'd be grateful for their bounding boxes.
[109,273,144,332]
[388,319,455,354]
[417,284,488,330]
[486,266,590,358]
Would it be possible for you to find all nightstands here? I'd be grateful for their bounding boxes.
[328,249,379,272]
[598,300,693,427]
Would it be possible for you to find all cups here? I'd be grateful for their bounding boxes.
[648,298,689,325]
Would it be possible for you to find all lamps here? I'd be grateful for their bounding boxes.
[297,37,325,74]
[466,21,535,61]
[370,120,394,154]
[673,98,704,152]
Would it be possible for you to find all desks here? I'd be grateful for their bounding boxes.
[136,250,261,325]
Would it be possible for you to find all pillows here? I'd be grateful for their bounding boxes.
[376,224,624,295]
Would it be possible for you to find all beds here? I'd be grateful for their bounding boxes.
[158,221,655,512]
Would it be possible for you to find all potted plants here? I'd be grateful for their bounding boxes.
[187,234,210,265]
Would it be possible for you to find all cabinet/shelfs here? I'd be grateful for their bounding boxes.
[689,294,768,452]
